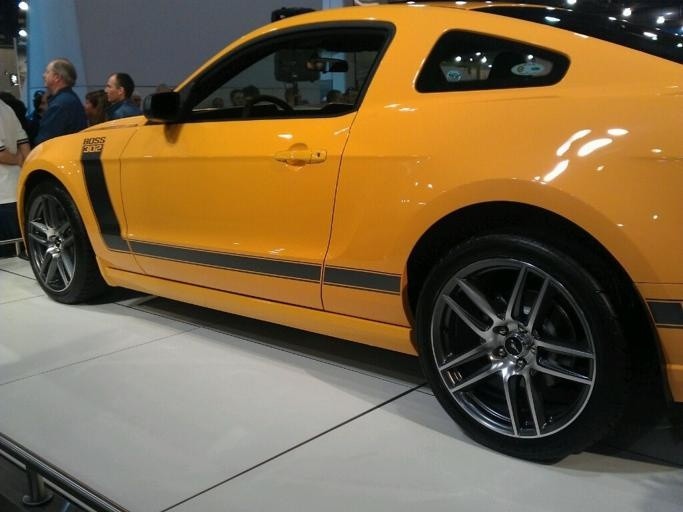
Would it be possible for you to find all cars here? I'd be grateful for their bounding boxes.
[18,6,682,463]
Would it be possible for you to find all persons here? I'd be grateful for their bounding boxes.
[212,84,360,109]
[1,58,145,255]
